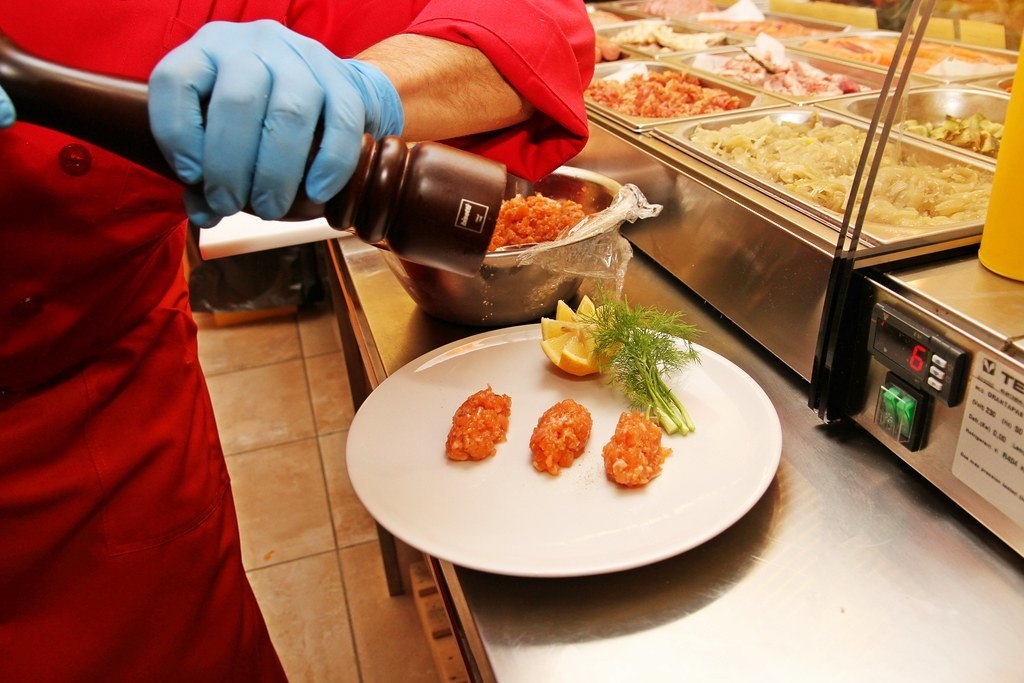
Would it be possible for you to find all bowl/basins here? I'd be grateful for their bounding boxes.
[583,0,1024,250]
[353,164,638,323]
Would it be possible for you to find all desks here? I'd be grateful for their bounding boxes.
[325,239,1023,683]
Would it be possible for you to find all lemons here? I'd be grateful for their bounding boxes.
[540,295,629,376]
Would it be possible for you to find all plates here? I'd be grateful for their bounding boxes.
[345,324,783,578]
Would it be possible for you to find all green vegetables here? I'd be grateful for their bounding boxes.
[573,281,709,437]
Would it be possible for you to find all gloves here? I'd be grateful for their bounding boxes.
[0,85,16,128]
[148,19,404,228]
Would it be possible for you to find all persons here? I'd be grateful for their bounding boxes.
[0,0,597,683]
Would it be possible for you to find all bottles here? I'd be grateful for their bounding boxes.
[977,31,1024,284]
